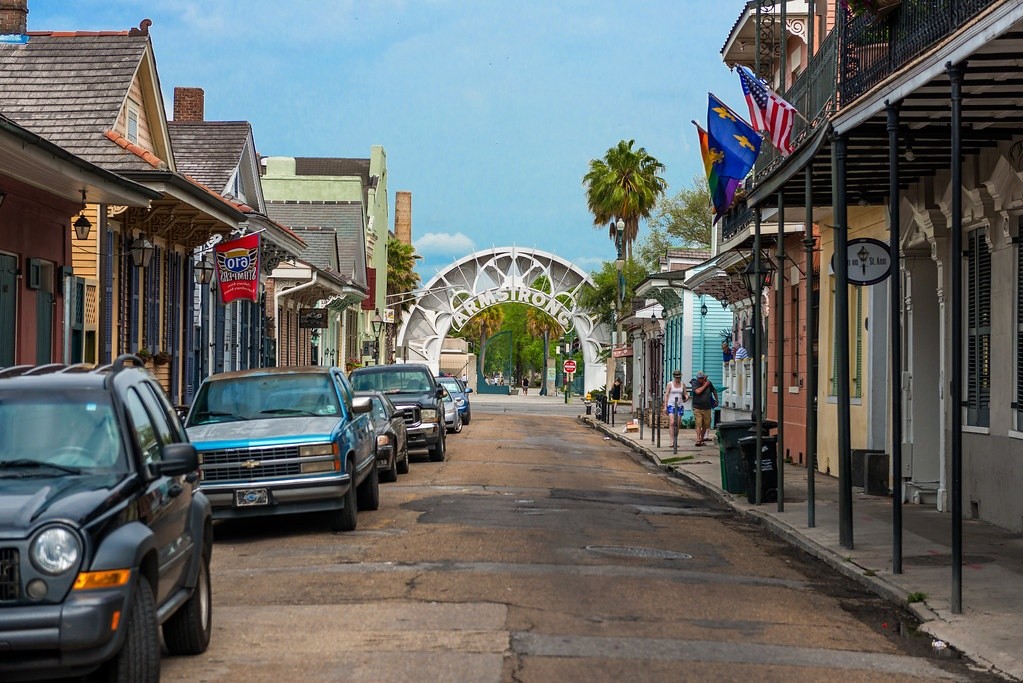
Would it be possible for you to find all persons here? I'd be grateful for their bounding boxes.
[500,377,504,385]
[494,375,497,385]
[462,374,466,387]
[612,378,622,413]
[731,342,749,359]
[692,371,718,445]
[490,378,495,384]
[664,370,688,446]
[722,341,733,364]
[522,377,529,395]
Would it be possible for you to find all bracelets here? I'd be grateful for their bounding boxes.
[716,400,719,402]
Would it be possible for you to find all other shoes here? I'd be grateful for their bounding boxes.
[695,439,707,446]
[614,410,617,414]
[668,443,680,447]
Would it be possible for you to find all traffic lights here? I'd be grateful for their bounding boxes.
[572,336,579,355]
[559,338,566,354]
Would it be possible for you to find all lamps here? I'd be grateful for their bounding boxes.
[640,318,646,341]
[661,289,668,319]
[192,245,214,284]
[127,207,154,267]
[650,290,656,327]
[701,302,708,319]
[629,323,635,343]
[720,294,732,312]
[72,189,93,240]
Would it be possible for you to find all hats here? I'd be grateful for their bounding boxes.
[697,374,708,379]
[672,371,681,377]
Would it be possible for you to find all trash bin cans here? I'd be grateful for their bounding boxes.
[737,434,777,504]
[716,428,728,491]
[716,418,778,494]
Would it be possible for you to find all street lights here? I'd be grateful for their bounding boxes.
[615,218,625,319]
[609,300,616,349]
[739,251,776,420]
[370,307,384,366]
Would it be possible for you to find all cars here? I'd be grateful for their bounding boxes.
[441,386,463,433]
[354,389,409,481]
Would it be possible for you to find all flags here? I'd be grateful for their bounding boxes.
[735,64,797,157]
[697,125,746,227]
[706,93,761,180]
[212,233,260,305]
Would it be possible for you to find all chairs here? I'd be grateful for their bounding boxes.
[407,379,422,390]
[359,381,374,391]
[266,394,298,409]
[297,394,330,406]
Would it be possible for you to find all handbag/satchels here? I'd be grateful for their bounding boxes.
[710,381,718,409]
[609,383,615,394]
[682,382,687,404]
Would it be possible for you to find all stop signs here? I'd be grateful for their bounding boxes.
[563,360,577,374]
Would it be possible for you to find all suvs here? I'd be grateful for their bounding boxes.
[347,362,447,462]
[420,375,473,425]
[0,352,215,683]
[174,367,380,530]
